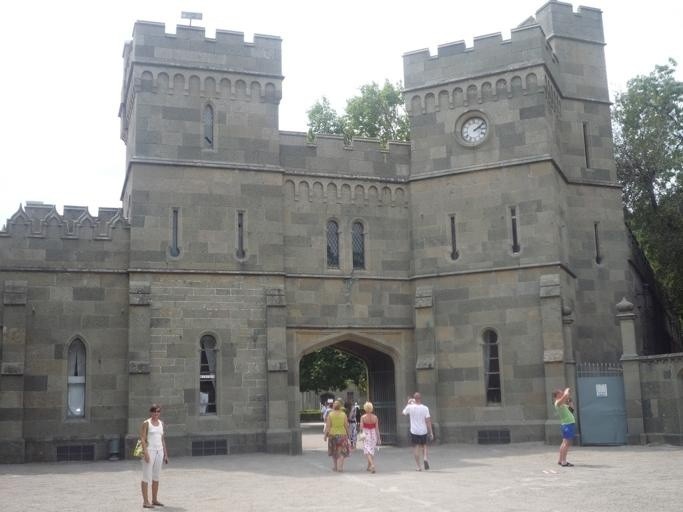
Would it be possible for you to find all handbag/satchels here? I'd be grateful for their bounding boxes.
[133,438,145,457]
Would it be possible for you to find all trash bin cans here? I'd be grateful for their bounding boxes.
[109,439,120,460]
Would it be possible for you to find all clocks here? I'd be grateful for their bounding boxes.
[461,116,487,144]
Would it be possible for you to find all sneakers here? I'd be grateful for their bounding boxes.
[142,502,153,507]
[151,500,163,506]
[556,459,574,467]
[415,458,429,470]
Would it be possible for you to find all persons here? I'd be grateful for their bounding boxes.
[553,387,577,467]
[402,392,434,471]
[323,401,351,473]
[359,402,381,474]
[320,397,360,450]
[140,404,168,508]
